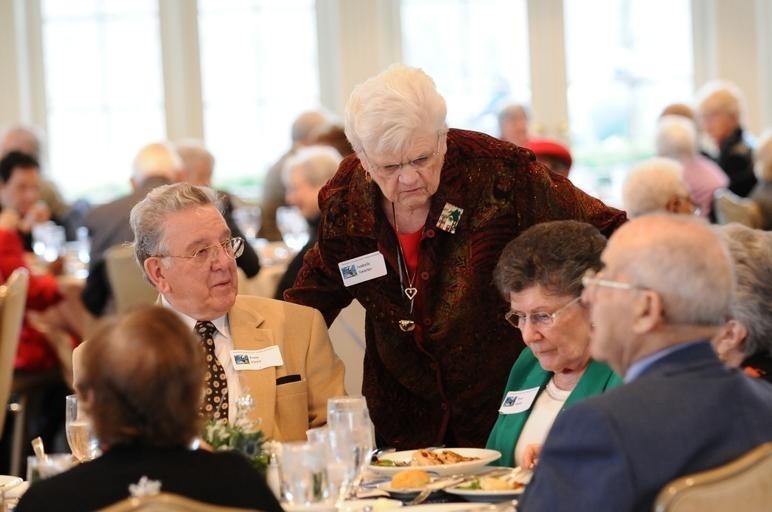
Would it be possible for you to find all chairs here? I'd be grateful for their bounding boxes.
[0,268,32,475]
[107,239,158,312]
[711,188,760,226]
[653,441,771,511]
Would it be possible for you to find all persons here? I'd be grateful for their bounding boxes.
[267,66,630,450]
[486,90,771,510]
[1,112,354,477]
[71,182,347,447]
[11,302,285,511]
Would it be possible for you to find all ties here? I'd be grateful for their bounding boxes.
[196,321,231,427]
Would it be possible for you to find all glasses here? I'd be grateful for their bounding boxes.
[504,298,579,330]
[361,134,444,180]
[161,237,246,267]
[582,266,634,293]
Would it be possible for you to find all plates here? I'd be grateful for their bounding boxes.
[371,447,501,476]
[377,481,428,497]
[442,483,523,502]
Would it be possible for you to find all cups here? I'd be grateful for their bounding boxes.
[65,393,98,462]
[27,454,71,489]
[264,393,374,512]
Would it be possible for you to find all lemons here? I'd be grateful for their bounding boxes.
[392,469,430,490]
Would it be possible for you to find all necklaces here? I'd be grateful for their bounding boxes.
[390,204,418,312]
